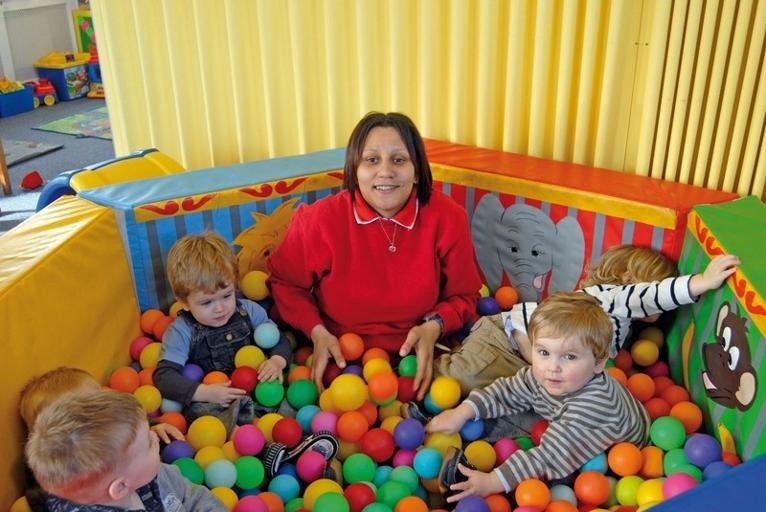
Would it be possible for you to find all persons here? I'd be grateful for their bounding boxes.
[152,230,340,478]
[24,389,229,512]
[16,367,102,434]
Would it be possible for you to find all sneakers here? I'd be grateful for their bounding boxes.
[259,440,287,478]
[400,400,437,425]
[439,445,478,491]
[280,429,340,465]
